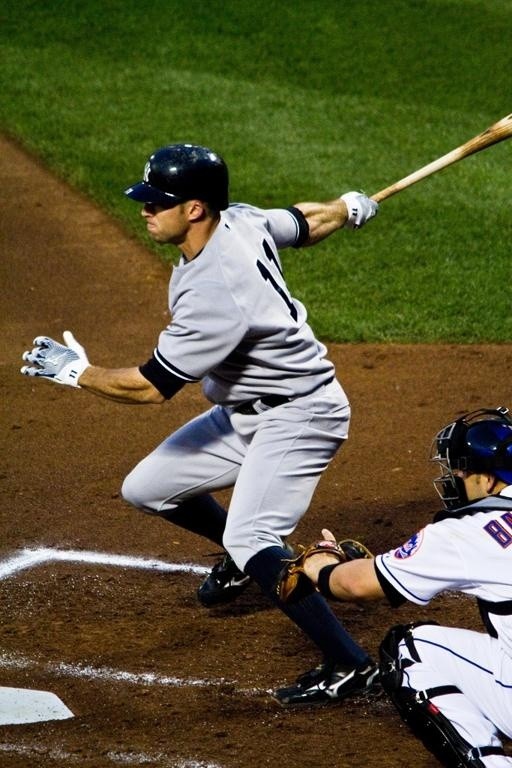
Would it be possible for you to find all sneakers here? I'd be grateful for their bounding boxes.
[269,658,384,708]
[196,547,255,611]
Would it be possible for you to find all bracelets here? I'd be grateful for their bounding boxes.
[318,561,340,600]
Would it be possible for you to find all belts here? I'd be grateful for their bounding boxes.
[230,374,338,420]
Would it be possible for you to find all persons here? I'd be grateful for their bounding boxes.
[18,141,386,708]
[301,404,511,768]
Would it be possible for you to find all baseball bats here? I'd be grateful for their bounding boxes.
[369,113,511,202]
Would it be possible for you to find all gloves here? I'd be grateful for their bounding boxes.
[338,187,380,229]
[20,329,90,392]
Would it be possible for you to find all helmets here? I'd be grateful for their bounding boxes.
[435,417,512,485]
[122,142,232,214]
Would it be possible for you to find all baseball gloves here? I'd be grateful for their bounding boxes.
[273,538,375,602]
